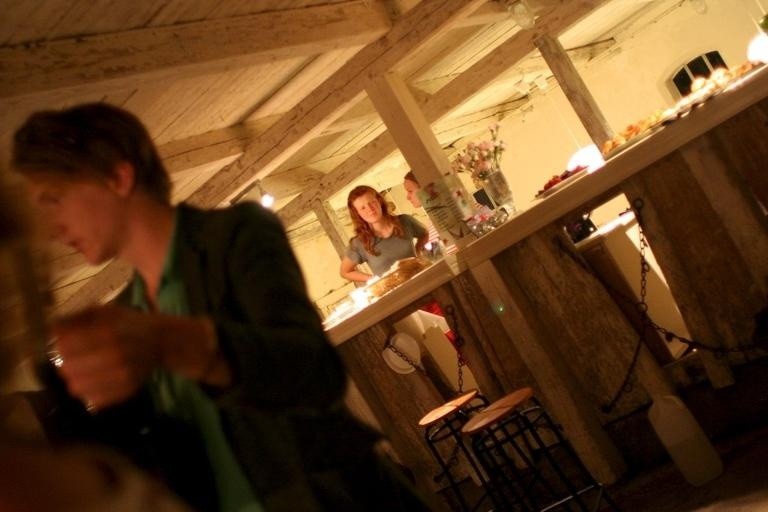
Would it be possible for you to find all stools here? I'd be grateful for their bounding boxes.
[459,386,622,512]
[417,387,541,512]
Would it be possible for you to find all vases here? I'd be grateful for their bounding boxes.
[477,169,520,218]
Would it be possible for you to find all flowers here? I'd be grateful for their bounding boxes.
[449,120,514,202]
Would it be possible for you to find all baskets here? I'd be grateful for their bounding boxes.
[366,253,433,299]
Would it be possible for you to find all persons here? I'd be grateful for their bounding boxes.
[338,183,457,343]
[7,98,409,512]
[402,170,425,210]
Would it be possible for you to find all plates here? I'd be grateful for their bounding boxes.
[601,125,655,161]
[534,165,590,201]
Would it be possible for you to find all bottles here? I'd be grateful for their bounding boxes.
[644,379,727,490]
